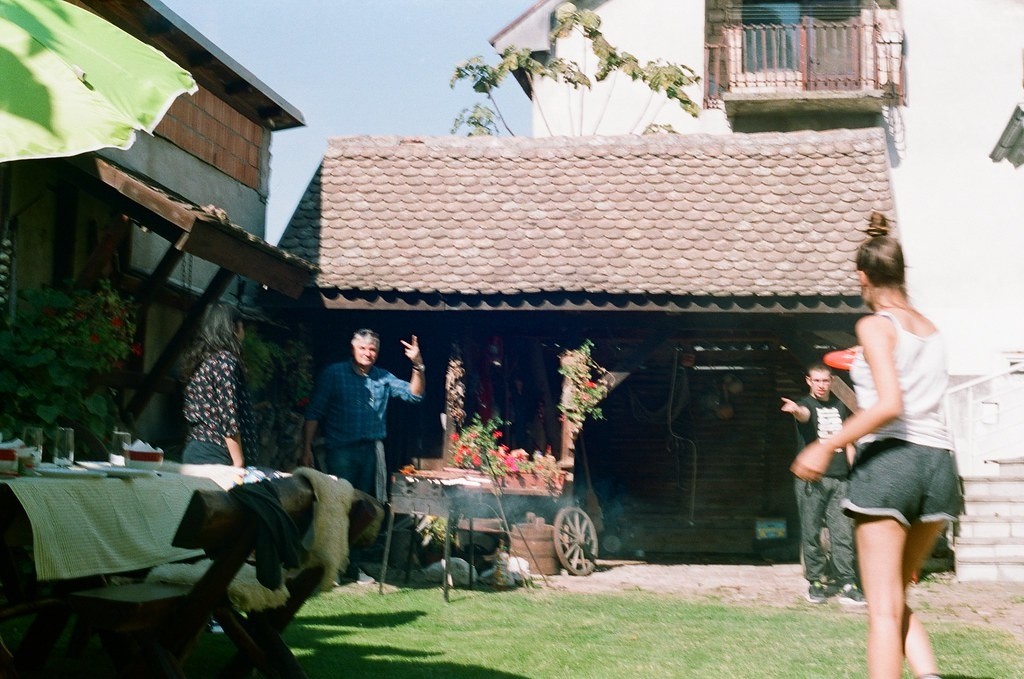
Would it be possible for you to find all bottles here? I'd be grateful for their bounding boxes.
[494,544,515,591]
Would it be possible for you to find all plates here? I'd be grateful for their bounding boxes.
[33,468,108,479]
[75,461,158,477]
[825,349,855,372]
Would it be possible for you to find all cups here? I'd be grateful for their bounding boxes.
[110,432,132,467]
[21,422,43,464]
[55,428,74,467]
[0,448,20,479]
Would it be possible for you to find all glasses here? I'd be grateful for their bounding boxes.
[359,330,379,338]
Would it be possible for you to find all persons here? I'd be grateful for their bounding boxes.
[304,330,426,584]
[792,212,962,679]
[181,303,260,634]
[780,363,869,606]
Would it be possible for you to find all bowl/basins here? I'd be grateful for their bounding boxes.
[123,451,165,470]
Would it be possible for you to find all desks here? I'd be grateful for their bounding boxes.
[0,460,258,674]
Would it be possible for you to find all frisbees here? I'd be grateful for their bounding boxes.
[821,347,855,370]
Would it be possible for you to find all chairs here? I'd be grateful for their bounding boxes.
[67,474,382,679]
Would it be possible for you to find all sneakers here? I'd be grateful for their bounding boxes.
[339,563,375,585]
[332,575,340,586]
[837,583,867,606]
[805,582,826,604]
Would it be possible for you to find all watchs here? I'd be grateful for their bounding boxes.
[412,364,425,373]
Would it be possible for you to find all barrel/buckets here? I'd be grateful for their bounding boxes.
[510,511,560,575]
